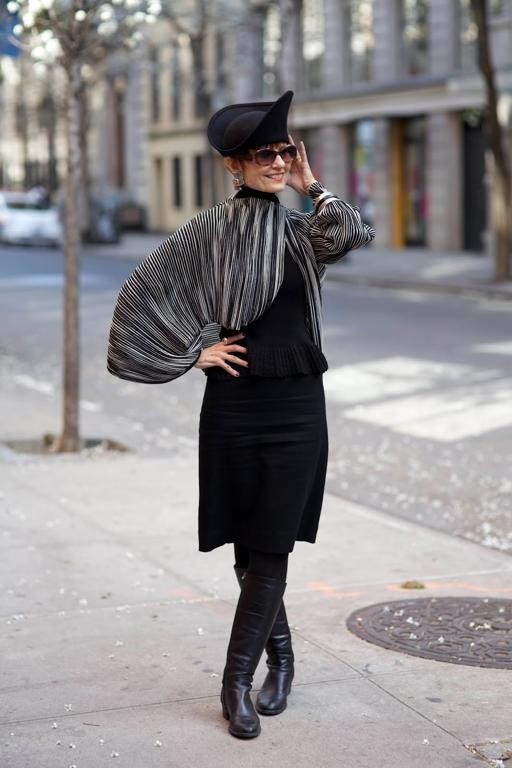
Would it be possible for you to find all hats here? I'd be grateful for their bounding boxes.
[204,89,298,159]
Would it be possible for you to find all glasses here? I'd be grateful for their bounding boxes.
[231,147,299,169]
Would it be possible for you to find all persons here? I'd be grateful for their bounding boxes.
[102,89,380,740]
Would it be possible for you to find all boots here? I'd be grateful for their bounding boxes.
[231,561,296,716]
[219,571,288,743]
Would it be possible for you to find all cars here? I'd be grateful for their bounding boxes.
[0,191,63,248]
